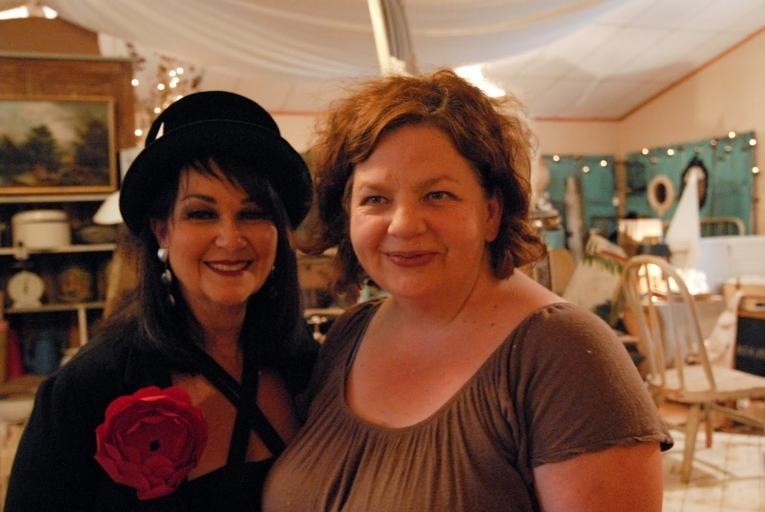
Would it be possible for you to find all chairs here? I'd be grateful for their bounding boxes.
[622,254,765,486]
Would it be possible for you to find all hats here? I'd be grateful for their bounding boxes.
[119,91,313,237]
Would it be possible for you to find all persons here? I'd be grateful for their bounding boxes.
[246,61,677,512]
[0,89,328,511]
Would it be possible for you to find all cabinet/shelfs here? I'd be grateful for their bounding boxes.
[1,198,122,393]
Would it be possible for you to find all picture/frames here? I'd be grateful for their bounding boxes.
[0,93,120,193]
